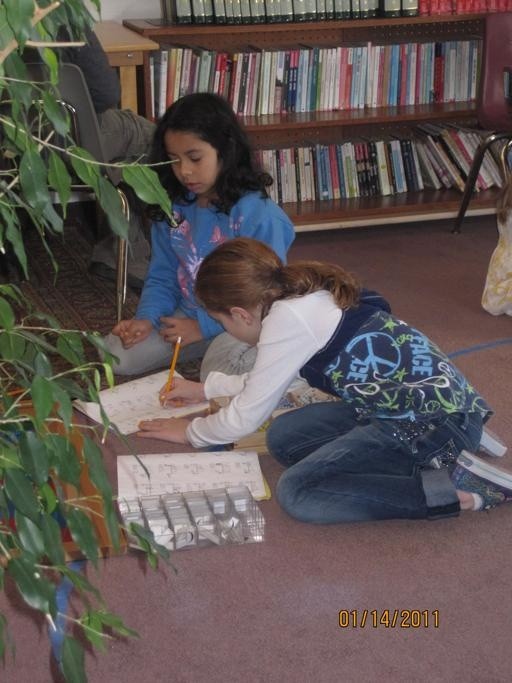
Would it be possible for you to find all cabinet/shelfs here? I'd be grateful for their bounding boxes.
[123,15,503,226]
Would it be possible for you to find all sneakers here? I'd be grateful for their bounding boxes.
[448,424,512,511]
[89,258,150,288]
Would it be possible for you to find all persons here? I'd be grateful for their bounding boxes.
[136,236,511,524]
[1,20,165,292]
[97,92,296,384]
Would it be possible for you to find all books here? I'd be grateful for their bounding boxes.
[150,32,483,121]
[117,450,272,503]
[245,121,504,206]
[171,2,511,27]
[111,484,267,555]
[69,367,210,435]
[220,385,340,449]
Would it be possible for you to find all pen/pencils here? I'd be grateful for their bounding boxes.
[163,336,182,409]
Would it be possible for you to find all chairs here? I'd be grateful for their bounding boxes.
[16,183,129,324]
[451,125,512,235]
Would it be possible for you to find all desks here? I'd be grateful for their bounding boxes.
[94,20,158,114]
